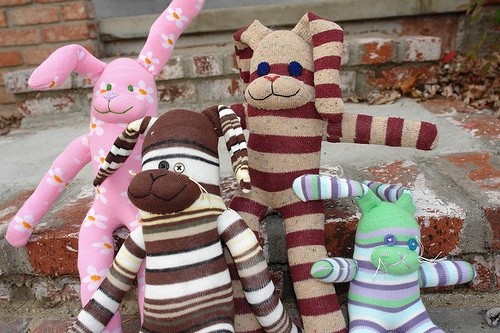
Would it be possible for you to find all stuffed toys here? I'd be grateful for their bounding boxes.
[4,1,206,332]
[219,12,439,333]
[292,172,479,333]
[65,104,304,333]
